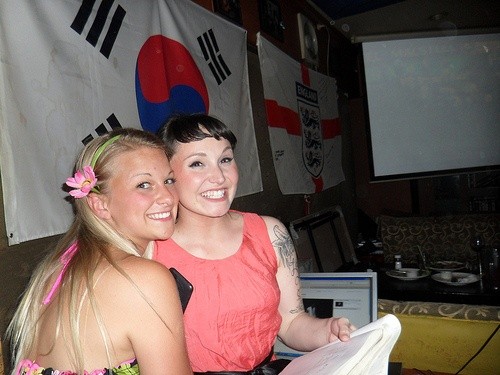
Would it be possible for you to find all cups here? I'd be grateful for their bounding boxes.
[441,270,452,281]
[406,270,418,278]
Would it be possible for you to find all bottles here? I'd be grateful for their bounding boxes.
[394,255,403,270]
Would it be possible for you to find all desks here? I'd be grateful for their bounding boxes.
[333,263,500,308]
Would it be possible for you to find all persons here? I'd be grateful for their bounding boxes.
[151,111,356,375]
[0,127,194,375]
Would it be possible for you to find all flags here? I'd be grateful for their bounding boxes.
[257,34,347,194]
[0,1,265,246]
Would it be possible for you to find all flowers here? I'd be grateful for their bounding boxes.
[65,167,100,199]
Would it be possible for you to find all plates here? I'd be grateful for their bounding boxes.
[432,273,479,286]
[428,261,466,272]
[386,270,430,280]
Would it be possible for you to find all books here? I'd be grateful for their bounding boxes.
[278,314,402,375]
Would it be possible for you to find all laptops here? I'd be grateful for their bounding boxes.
[273,271,377,360]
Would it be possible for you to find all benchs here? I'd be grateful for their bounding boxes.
[380,215,500,266]
[377,299,500,375]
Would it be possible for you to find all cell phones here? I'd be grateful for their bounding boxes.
[169,267,193,314]
